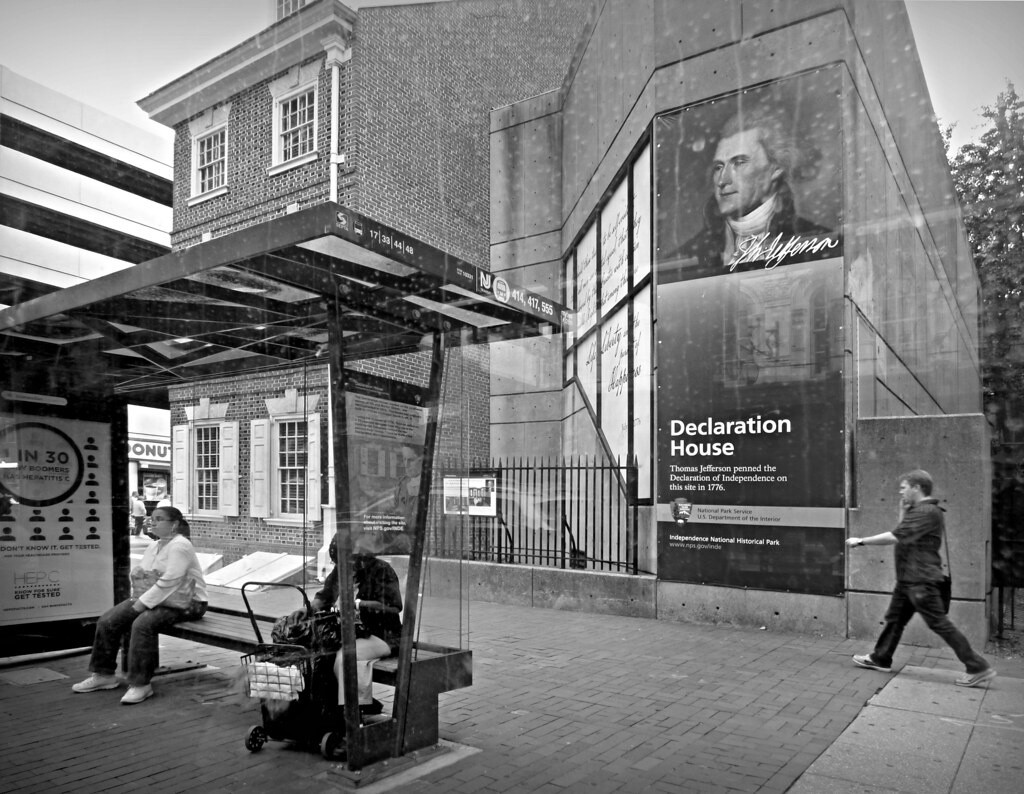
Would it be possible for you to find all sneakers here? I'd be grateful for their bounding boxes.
[120,684,154,703]
[954,668,997,687]
[71,673,119,692]
[852,653,892,672]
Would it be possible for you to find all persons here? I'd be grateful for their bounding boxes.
[844,468,997,688]
[385,433,425,555]
[130,492,147,535]
[310,529,403,705]
[156,494,171,507]
[72,506,209,703]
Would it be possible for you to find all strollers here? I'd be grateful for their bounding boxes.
[241,581,342,758]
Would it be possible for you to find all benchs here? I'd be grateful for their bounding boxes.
[160,610,474,753]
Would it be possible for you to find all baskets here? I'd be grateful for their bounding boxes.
[241,647,306,701]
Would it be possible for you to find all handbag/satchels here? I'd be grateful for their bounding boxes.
[938,576,951,614]
[131,569,197,610]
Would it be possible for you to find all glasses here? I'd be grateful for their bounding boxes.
[149,516,174,523]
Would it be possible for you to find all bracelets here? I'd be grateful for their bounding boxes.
[858,539,865,546]
